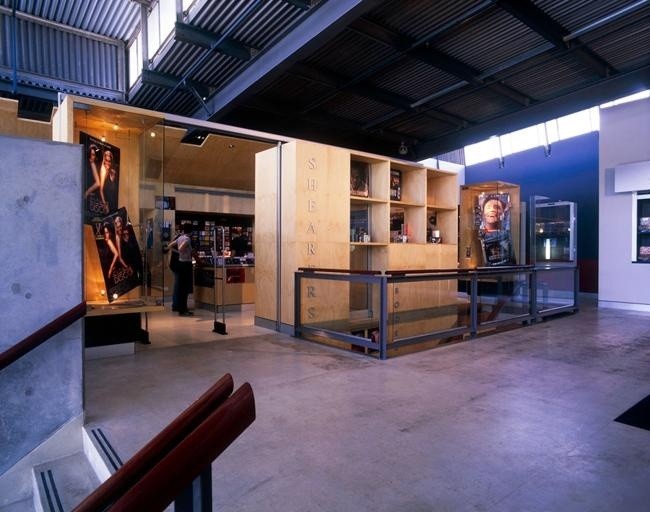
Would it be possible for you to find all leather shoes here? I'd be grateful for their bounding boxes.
[179,311,194,316]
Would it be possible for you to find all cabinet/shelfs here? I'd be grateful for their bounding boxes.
[349,148,459,322]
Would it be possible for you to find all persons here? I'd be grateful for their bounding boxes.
[86,143,117,215]
[230,229,246,257]
[480,194,506,232]
[101,215,143,280]
[167,223,194,317]
